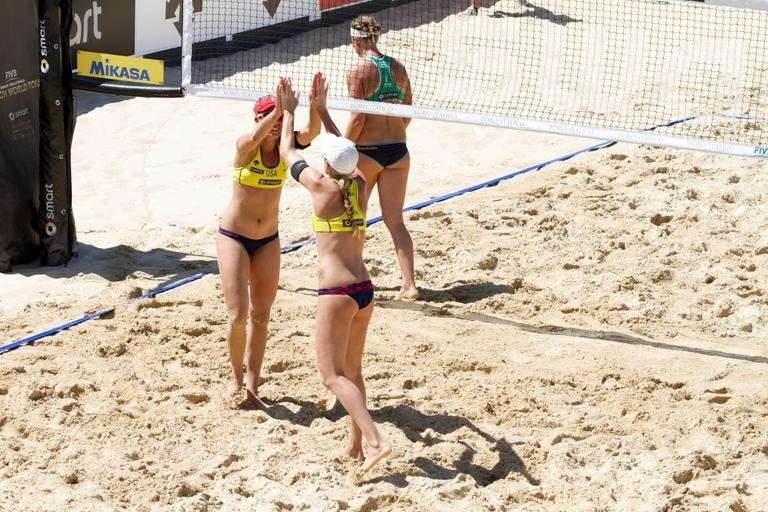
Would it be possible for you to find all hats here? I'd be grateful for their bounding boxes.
[254,94,275,113]
[320,133,359,174]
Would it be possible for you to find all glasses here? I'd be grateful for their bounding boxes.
[257,111,283,122]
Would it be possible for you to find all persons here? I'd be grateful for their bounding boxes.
[215,73,327,410]
[342,14,419,299]
[278,70,392,484]
